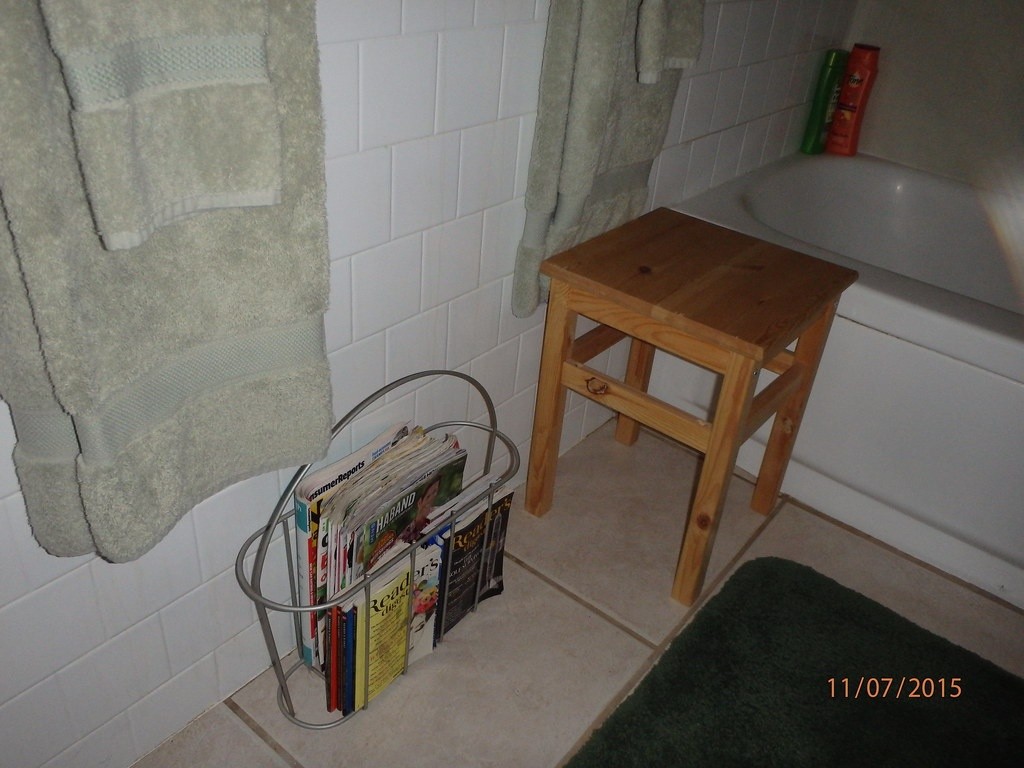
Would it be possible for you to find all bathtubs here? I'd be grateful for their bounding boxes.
[651,145,1024,615]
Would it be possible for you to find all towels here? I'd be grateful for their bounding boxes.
[509,4,705,317]
[1,2,330,562]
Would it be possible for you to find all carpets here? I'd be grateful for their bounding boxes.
[557,555,1024,768]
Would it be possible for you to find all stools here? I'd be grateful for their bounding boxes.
[524,206,861,607]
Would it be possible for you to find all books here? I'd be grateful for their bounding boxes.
[293,423,516,718]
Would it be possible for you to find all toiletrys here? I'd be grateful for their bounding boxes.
[802,50,855,153]
[827,43,880,155]
